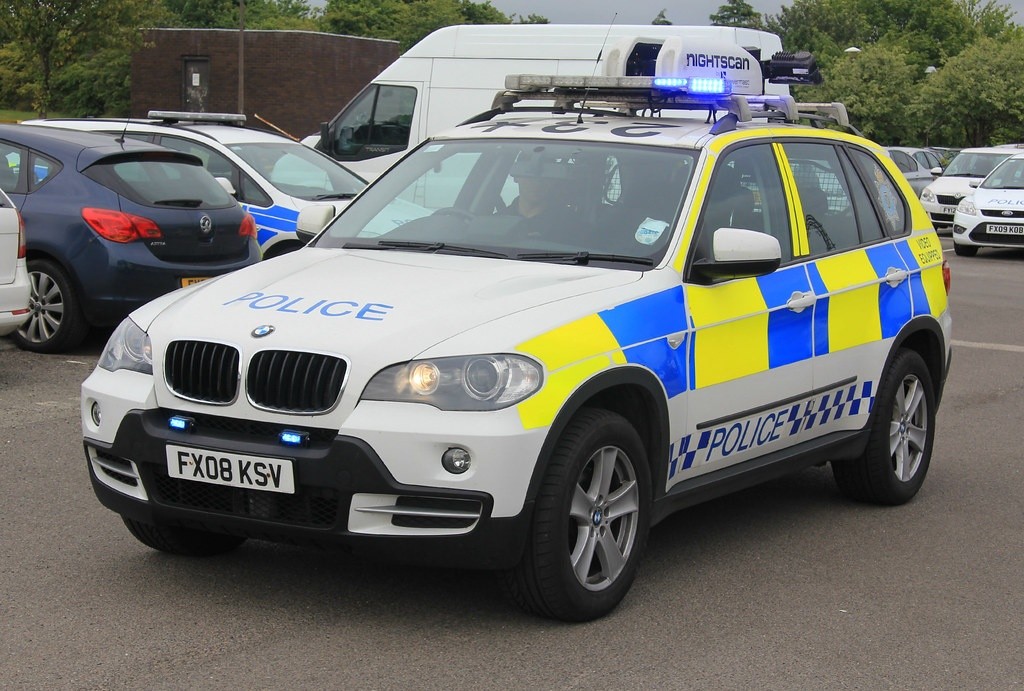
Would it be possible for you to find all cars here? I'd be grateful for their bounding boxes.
[7,110,436,262]
[953,152,1024,256]
[810,146,937,216]
[0,187,31,338]
[0,124,265,356]
[919,143,1024,231]
[899,146,964,180]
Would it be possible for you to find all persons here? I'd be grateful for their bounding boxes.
[486,153,565,240]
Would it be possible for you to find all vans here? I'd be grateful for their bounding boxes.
[270,22,791,218]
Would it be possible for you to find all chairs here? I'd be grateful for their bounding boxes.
[801,187,842,254]
[972,158,993,175]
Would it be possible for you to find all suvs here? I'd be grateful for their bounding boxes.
[79,69,968,629]
